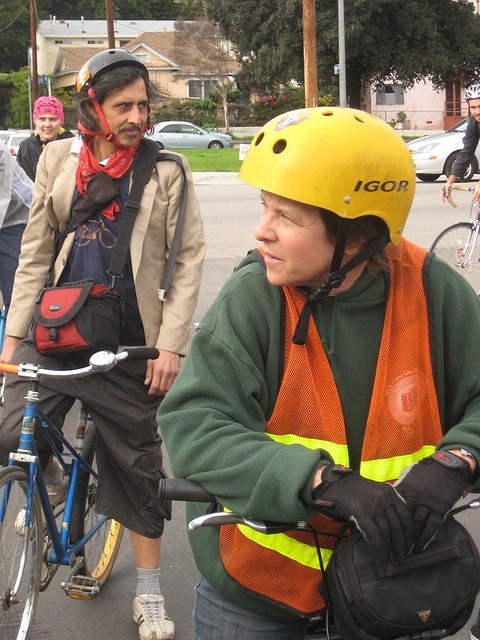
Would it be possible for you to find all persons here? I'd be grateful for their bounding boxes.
[0,49,207,640]
[155,102,477,637]
[442,89,477,241]
[1,97,78,310]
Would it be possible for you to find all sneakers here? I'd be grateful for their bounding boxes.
[14,469,72,537]
[132,594,176,639]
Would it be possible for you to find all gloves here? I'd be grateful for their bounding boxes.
[392,452,471,554]
[314,461,417,562]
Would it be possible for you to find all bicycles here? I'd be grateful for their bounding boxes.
[158,479,480,640]
[1,347,160,640]
[429,183,480,297]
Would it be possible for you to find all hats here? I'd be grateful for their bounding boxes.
[33,96,64,125]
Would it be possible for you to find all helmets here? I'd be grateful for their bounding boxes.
[465,84,480,100]
[77,48,153,148]
[238,106,417,346]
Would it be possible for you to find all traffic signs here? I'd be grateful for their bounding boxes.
[28,75,49,84]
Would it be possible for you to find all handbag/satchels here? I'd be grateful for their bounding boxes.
[326,517,480,640]
[32,138,160,359]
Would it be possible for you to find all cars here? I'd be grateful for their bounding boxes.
[0,130,15,144]
[407,116,480,183]
[5,134,31,159]
[144,121,235,150]
